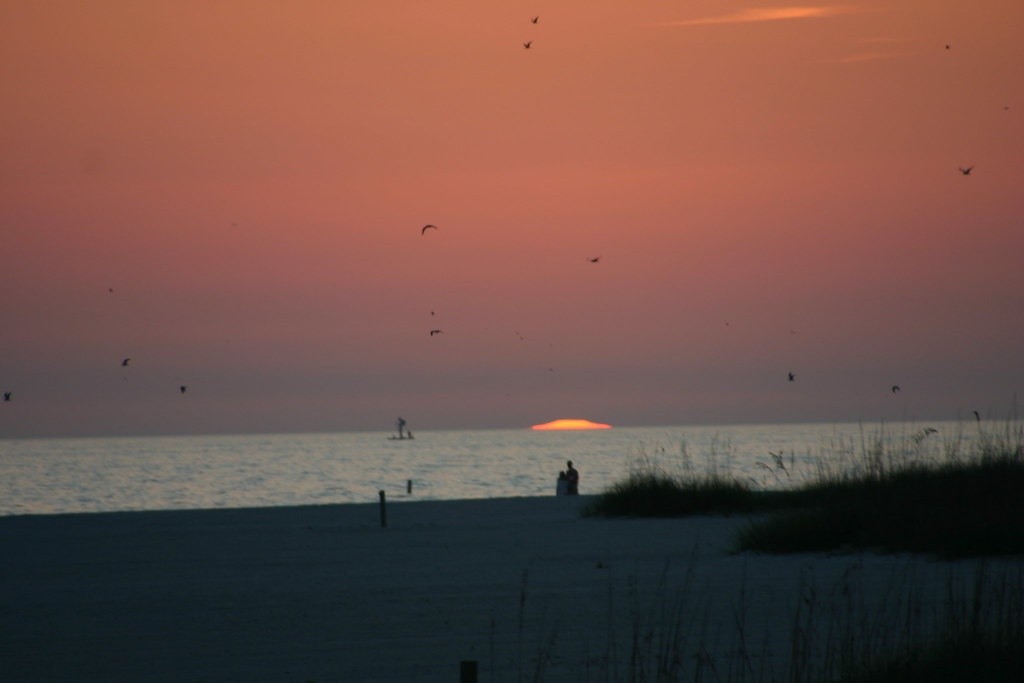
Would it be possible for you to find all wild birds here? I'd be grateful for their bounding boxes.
[422,224,438,236]
[2,253,989,395]
[942,41,1013,179]
[524,16,539,49]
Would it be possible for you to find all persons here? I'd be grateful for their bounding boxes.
[398,417,405,438]
[557,460,579,495]
